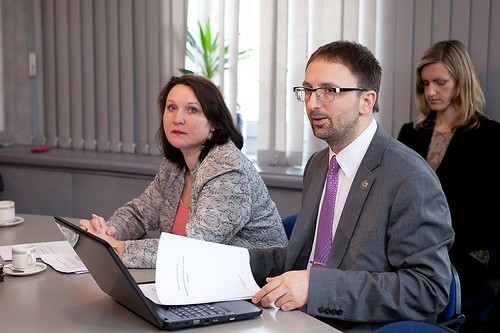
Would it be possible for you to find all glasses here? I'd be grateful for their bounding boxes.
[293,86,368,103]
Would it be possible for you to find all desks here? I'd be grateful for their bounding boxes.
[0,213,342,333]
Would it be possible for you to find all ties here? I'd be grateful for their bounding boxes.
[298,156,340,314]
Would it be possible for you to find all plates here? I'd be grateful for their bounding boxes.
[2,262,47,275]
[0,217,24,226]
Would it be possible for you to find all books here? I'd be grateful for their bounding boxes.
[0,241,90,275]
[138,232,262,305]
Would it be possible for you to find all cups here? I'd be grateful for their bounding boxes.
[12,244,36,272]
[0,200,16,223]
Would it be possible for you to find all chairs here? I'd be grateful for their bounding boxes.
[280,214,466,333]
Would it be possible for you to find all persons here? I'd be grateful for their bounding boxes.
[395,39,500,333]
[248,40,456,333]
[77,76,288,269]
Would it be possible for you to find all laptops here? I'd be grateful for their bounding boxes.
[53,215,262,331]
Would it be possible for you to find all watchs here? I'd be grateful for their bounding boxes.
[112,239,122,254]
